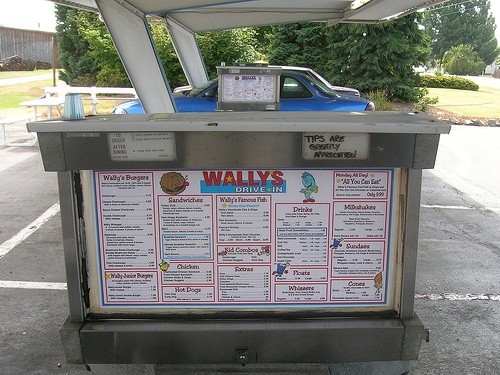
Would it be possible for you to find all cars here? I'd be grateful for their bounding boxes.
[111,60,375,114]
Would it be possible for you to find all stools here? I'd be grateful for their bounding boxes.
[0,118,16,146]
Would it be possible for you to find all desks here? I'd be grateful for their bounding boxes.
[20,97,68,146]
